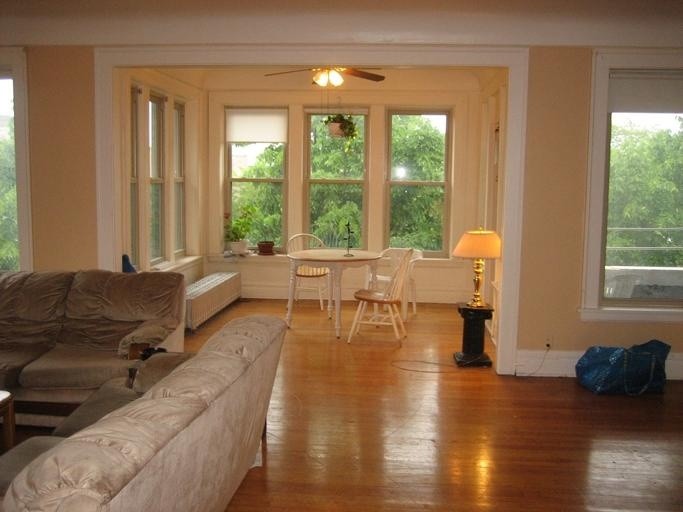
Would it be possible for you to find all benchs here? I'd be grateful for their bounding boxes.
[184,271,242,333]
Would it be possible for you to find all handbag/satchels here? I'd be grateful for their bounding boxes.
[575,339,671,396]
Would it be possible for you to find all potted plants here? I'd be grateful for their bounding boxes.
[320,111,358,142]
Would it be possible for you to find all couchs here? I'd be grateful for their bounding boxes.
[0,314,290,511]
[0,270,186,427]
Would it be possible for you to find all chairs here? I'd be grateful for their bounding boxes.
[287,233,332,309]
[367,246,423,322]
[346,248,413,342]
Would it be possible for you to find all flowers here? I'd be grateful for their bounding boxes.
[224,204,254,242]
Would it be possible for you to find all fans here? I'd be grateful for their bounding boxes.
[263,65,385,86]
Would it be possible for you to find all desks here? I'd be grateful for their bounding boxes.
[454,302,494,367]
[0,390,18,448]
[285,250,381,339]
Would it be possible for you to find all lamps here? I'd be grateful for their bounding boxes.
[314,70,344,87]
[452,225,502,307]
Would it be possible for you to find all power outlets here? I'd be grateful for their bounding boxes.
[544,335,552,350]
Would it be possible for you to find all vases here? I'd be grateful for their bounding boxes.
[256,241,276,255]
[229,240,250,255]
